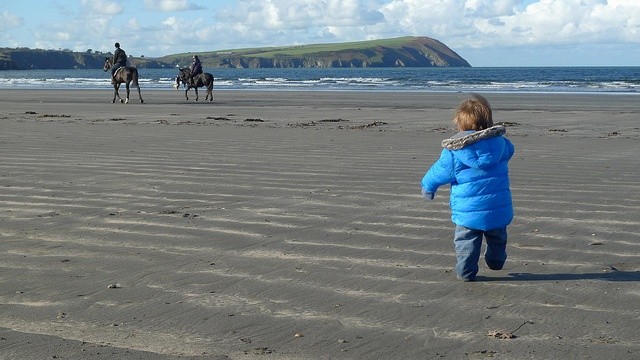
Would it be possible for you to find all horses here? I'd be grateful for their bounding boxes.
[103,56,144,104]
[179,66,214,102]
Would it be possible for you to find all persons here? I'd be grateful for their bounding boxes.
[175,74,181,89]
[109,42,127,84]
[421,93,515,281]
[187,55,203,87]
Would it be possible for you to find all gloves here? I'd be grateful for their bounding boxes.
[421,187,435,201]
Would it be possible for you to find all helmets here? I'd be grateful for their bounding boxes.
[192,55,198,60]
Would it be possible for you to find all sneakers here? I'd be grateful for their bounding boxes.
[456,274,475,283]
